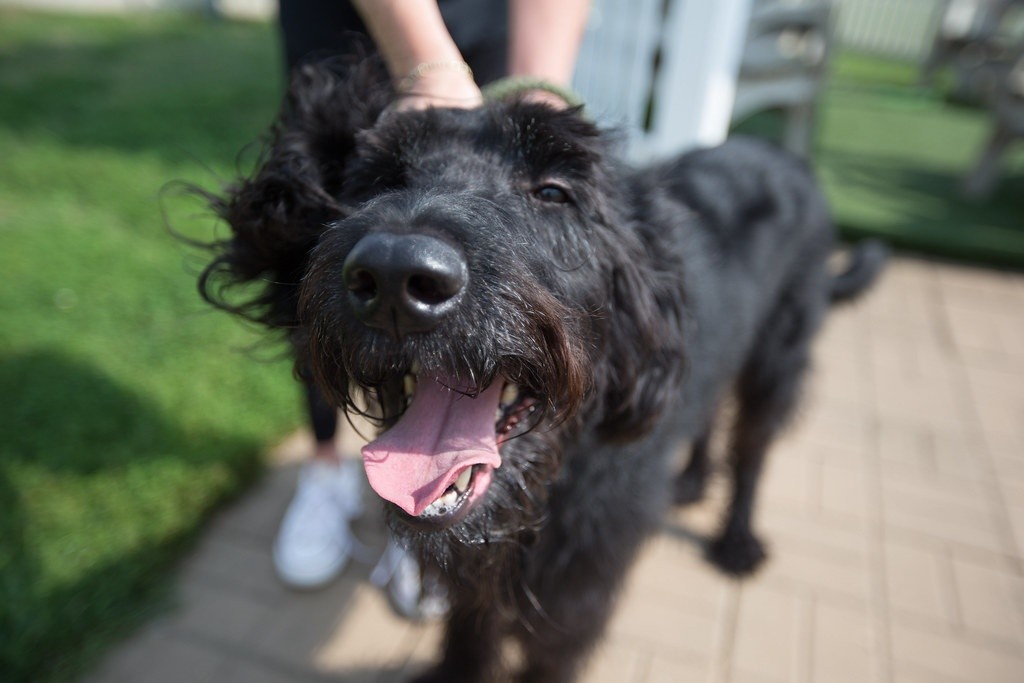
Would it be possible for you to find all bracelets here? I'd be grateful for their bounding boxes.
[396,60,474,95]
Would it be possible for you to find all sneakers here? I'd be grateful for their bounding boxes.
[272,457,446,623]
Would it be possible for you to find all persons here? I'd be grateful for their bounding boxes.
[275,0,592,621]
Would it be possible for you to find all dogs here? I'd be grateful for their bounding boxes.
[159,28,887,683]
